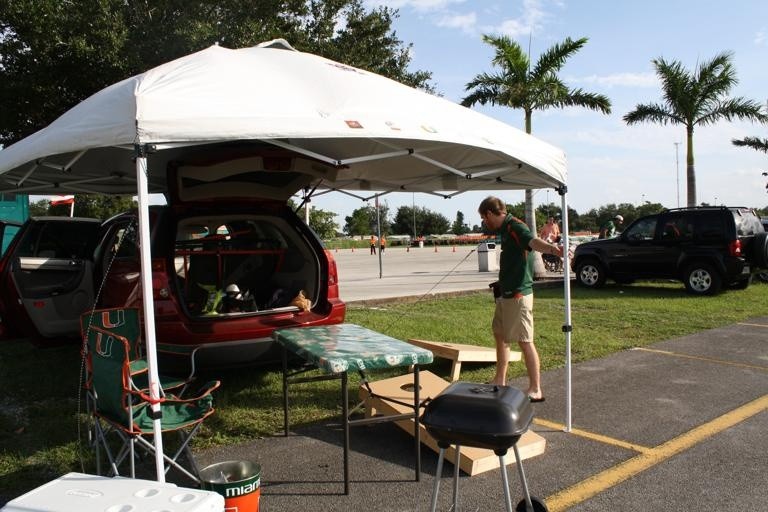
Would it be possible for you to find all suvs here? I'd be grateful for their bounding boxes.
[571,206,768,296]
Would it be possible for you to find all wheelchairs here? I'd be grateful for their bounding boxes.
[542,253,564,274]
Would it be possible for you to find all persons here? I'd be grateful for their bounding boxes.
[381,235,385,252]
[370,236,376,255]
[541,216,561,271]
[599,214,624,239]
[478,196,575,402]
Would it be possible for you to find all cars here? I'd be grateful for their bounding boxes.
[0,142,347,385]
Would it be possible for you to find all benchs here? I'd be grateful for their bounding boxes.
[78,306,222,493]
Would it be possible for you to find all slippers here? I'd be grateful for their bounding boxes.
[526,392,545,402]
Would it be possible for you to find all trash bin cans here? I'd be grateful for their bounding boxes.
[478,242,497,272]
[419,241,423,248]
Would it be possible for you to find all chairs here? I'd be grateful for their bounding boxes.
[186,233,276,299]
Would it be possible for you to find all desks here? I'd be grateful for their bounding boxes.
[273,324,433,496]
